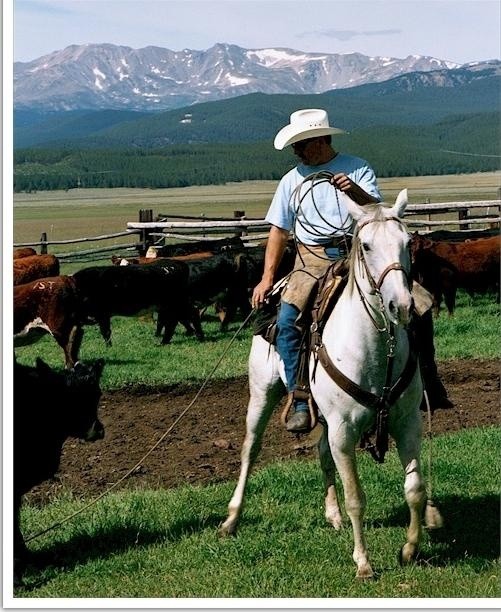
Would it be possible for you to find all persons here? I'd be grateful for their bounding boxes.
[246,106,455,437]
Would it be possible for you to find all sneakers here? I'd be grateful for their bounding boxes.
[284,401,313,432]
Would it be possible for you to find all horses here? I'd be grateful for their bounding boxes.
[216,186,427,585]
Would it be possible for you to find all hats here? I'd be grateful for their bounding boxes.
[272,107,346,154]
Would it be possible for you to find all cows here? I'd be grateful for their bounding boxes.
[13,356,107,591]
[13,235,300,371]
[406,226,501,319]
